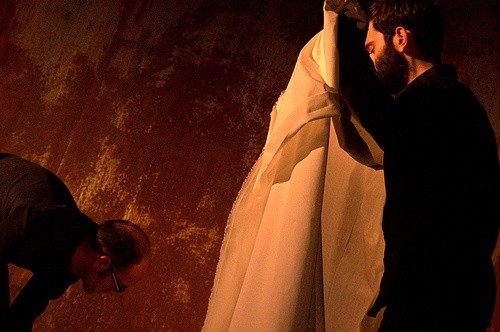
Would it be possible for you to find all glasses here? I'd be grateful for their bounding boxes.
[110,261,125,292]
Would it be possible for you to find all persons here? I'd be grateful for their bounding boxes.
[336,0,499,332]
[0,152,152,332]
